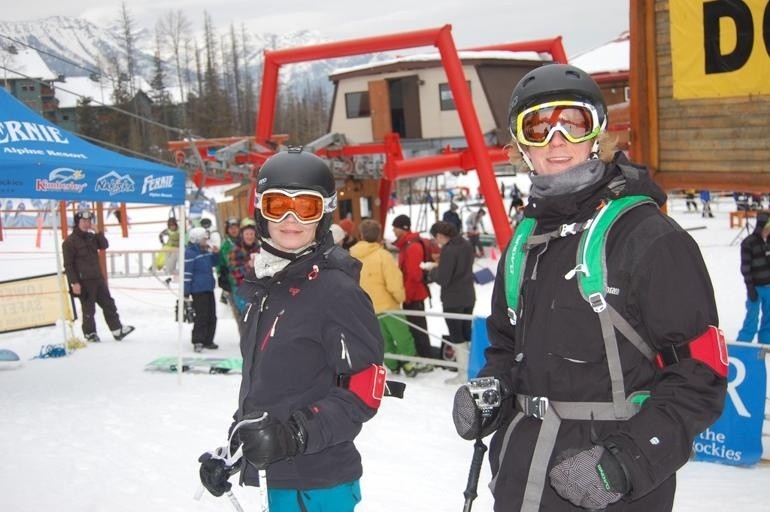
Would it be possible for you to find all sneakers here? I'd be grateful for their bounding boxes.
[206,341,220,350]
[403,360,433,379]
[111,324,135,342]
[85,332,101,343]
[193,342,204,351]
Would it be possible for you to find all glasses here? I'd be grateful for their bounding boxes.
[258,189,328,225]
[514,99,605,147]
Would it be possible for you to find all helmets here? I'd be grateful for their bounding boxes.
[189,227,207,243]
[507,61,611,150]
[73,211,95,223]
[240,217,257,230]
[224,216,241,235]
[252,147,339,244]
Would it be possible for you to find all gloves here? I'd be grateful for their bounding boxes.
[237,409,302,473]
[548,440,629,511]
[451,383,509,441]
[199,446,238,499]
[748,289,758,302]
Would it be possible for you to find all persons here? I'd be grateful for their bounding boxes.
[736,209,769,347]
[196,145,388,510]
[700,191,714,219]
[60,208,136,348]
[683,188,699,214]
[449,63,735,511]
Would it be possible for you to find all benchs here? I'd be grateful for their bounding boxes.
[729,208,770,229]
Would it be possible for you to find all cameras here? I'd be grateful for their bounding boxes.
[467,377,501,410]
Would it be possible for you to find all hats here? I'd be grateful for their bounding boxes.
[392,214,411,231]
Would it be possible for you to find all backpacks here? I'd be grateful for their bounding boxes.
[403,237,439,285]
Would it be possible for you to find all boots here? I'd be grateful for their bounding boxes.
[443,339,470,387]
